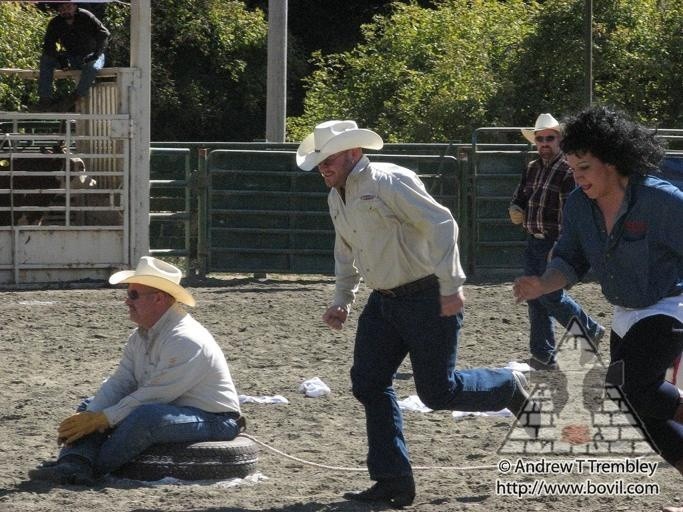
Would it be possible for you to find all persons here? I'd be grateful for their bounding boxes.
[508,110,605,373]
[28,2,114,114]
[27,254,248,490]
[292,117,543,506]
[510,103,683,482]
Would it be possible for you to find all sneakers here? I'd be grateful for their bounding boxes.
[28,460,97,488]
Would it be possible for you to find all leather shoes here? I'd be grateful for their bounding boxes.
[504,362,542,440]
[508,358,560,374]
[343,479,416,507]
[579,324,607,366]
[36,460,105,483]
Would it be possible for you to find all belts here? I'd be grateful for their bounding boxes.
[531,233,547,240]
[220,412,247,431]
[377,275,439,299]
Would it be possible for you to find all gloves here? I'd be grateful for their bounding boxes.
[509,204,524,225]
[58,412,110,444]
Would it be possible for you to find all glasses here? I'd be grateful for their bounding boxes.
[317,153,339,167]
[126,289,160,300]
[534,135,558,144]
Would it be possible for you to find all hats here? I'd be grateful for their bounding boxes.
[108,256,196,307]
[295,119,384,170]
[520,113,561,146]
